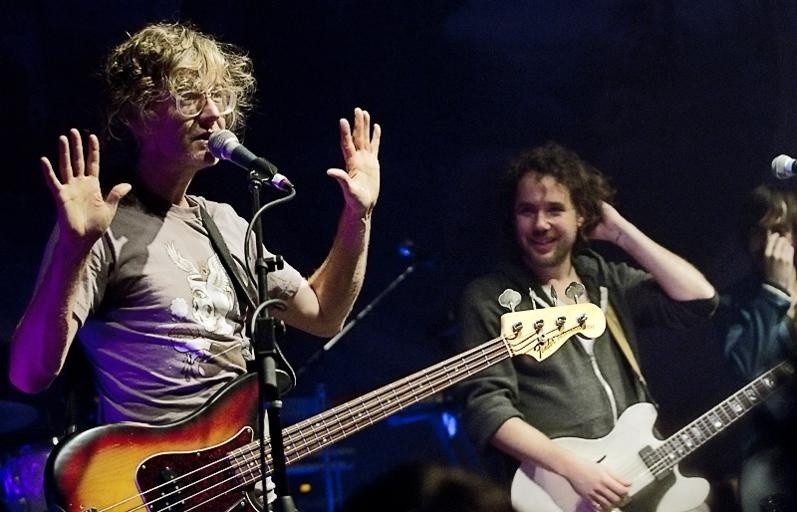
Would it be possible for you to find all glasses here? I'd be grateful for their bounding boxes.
[170,90,238,119]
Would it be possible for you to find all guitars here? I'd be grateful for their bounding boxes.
[511,360,792,512]
[43,280,608,512]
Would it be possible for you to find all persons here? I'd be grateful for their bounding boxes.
[7,22,386,434]
[679,155,796,511]
[452,142,720,508]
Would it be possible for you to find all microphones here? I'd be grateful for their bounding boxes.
[208,128,294,194]
[770,153,797,181]
